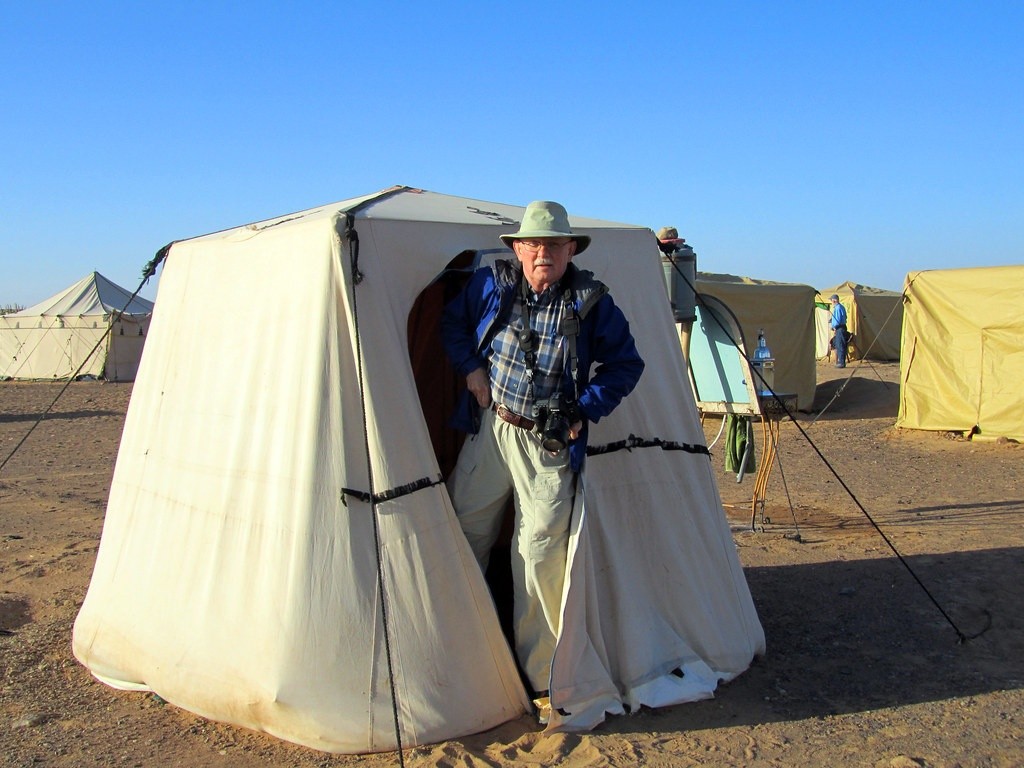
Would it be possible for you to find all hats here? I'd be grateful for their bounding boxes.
[827,295,839,299]
[499,201,591,256]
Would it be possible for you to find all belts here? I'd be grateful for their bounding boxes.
[491,402,535,431]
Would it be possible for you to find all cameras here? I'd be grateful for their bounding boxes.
[531,391,582,451]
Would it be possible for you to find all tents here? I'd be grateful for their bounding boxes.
[71,183,767,753]
[667,271,822,415]
[0,271,155,383]
[815,280,905,363]
[892,266,1024,443]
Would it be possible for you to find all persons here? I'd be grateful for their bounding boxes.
[444,200,645,726]
[828,294,847,368]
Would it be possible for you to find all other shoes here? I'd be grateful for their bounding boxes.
[835,364,846,368]
[536,690,551,720]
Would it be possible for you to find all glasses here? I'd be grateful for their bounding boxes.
[518,240,571,253]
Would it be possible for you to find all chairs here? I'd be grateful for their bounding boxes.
[829,331,853,363]
